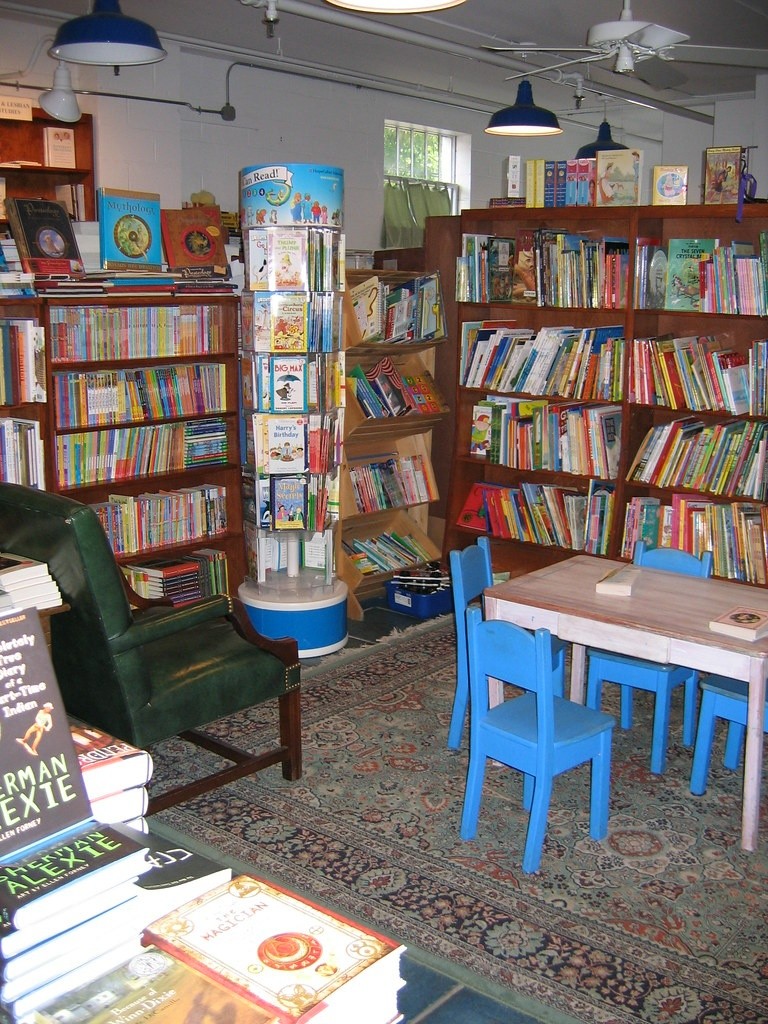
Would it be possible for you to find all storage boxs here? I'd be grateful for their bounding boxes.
[386,580,450,618]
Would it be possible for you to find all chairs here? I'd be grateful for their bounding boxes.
[586,538,711,774]
[0,483,302,819]
[450,538,564,750]
[691,674,768,796]
[459,606,616,872]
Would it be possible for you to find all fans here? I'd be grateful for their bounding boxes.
[483,4,768,82]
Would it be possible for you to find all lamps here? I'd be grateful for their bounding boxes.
[22,35,83,121]
[48,0,167,66]
[575,100,628,160]
[484,66,564,137]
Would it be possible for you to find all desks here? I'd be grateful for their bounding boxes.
[482,554,768,854]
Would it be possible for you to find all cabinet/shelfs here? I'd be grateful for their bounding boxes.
[451,207,629,577]
[0,296,53,494]
[44,294,246,621]
[339,268,452,622]
[237,164,341,588]
[615,203,768,585]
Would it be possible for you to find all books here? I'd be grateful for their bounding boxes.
[595,559,641,596]
[44,127,76,167]
[594,149,643,207]
[244,226,448,583]
[705,146,741,204]
[455,226,768,583]
[0,184,239,608]
[710,605,768,643]
[652,165,688,205]
[0,550,407,1024]
